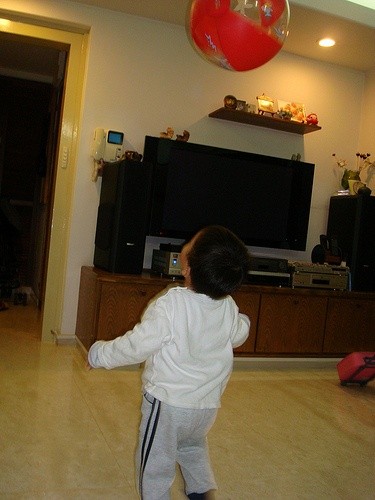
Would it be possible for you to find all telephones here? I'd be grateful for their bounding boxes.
[93,129,125,163]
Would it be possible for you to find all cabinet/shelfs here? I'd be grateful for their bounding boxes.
[74,266,375,362]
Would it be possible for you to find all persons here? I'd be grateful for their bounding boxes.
[87,225,250,500]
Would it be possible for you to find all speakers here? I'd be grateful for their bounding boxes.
[94,158,148,276]
[327,194,375,292]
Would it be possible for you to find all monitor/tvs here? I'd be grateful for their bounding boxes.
[142,136,316,253]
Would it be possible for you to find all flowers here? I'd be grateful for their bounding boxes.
[355,152,371,173]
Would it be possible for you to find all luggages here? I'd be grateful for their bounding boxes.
[336,352,375,386]
